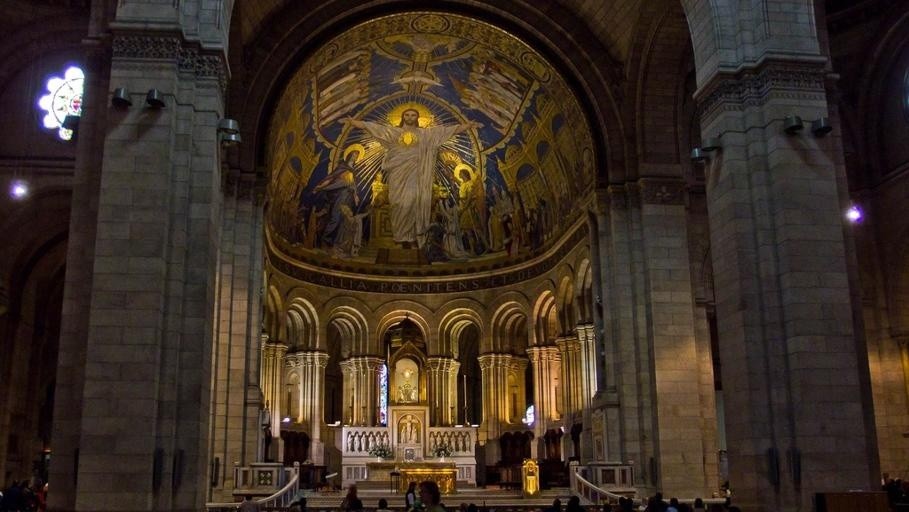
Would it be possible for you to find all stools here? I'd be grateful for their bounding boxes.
[390,472,400,493]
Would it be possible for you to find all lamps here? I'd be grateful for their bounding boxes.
[216,116,243,148]
[782,112,832,138]
[688,136,721,163]
[110,84,165,110]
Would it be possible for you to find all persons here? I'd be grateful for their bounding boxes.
[881,472,909,508]
[305,109,549,259]
[0,479,48,512]
[241,481,730,512]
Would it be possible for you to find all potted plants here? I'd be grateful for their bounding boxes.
[367,440,392,462]
[431,442,454,462]
